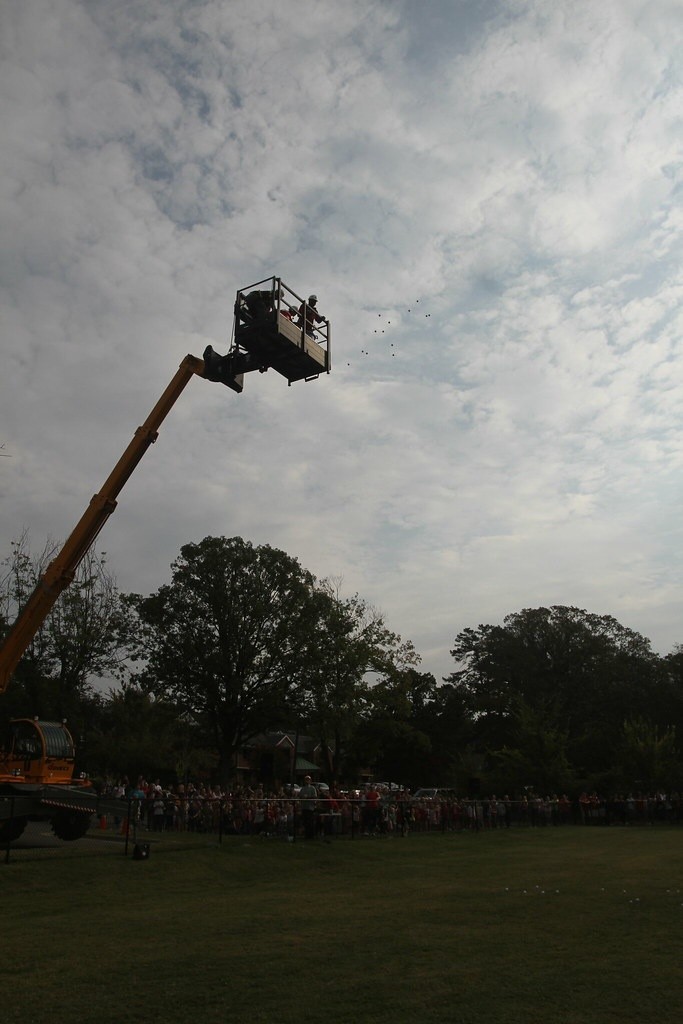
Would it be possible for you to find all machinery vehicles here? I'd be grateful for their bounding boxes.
[0,275,332,842]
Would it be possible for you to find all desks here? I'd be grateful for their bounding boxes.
[319,812,342,840]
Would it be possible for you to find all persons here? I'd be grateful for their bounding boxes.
[245,289,284,320]
[298,776,317,838]
[97,776,683,844]
[280,305,298,322]
[296,295,325,337]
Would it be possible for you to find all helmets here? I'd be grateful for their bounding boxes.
[276,289,284,297]
[305,775,312,780]
[289,306,298,313]
[308,294,318,301]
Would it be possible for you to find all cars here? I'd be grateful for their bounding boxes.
[285,782,458,799]
